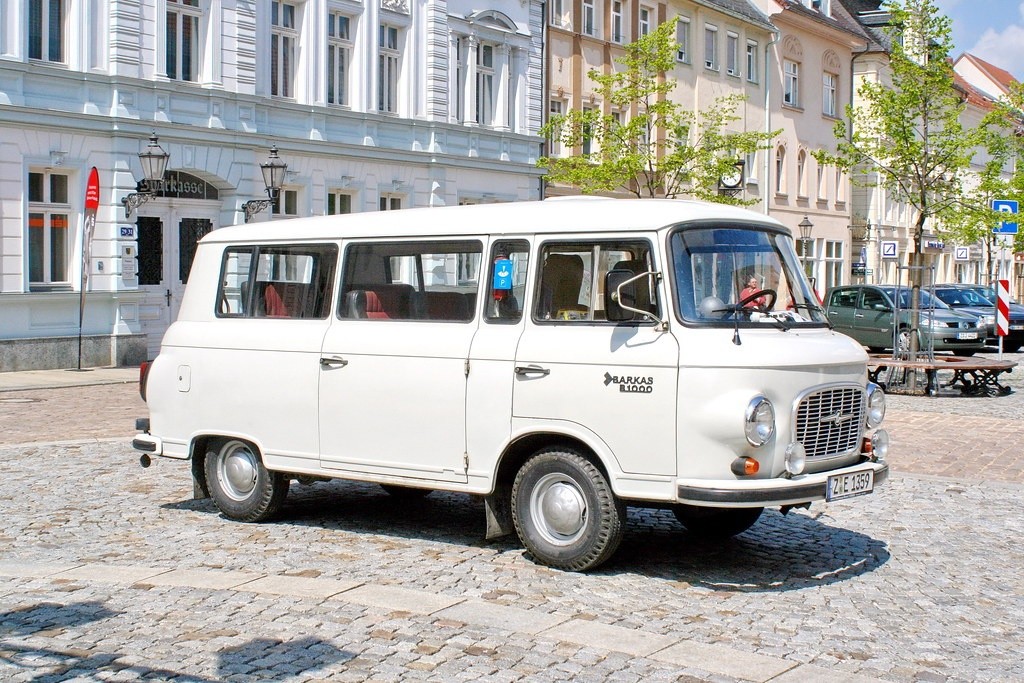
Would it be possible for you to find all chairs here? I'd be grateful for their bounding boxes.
[241,281,316,317]
[415,293,470,320]
[344,284,417,319]
[614,260,655,320]
[465,293,518,321]
[538,255,597,319]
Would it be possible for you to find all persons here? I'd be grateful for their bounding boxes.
[739,276,766,309]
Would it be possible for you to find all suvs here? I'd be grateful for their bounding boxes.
[821,284,988,358]
[887,283,1024,352]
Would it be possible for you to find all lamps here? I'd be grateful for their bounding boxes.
[121,128,171,219]
[241,140,289,222]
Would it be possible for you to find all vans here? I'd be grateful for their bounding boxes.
[132,195,890,572]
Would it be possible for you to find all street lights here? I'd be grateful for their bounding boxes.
[797,213,814,273]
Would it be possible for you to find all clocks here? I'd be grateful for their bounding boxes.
[722,165,743,187]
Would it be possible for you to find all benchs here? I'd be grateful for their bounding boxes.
[867,353,1018,397]
[840,295,856,307]
